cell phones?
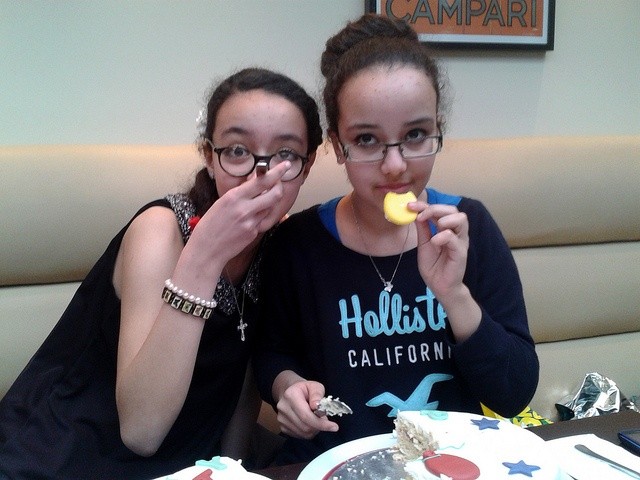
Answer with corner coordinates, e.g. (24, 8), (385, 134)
(617, 427), (640, 452)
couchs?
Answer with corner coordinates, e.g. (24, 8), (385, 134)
(0, 134), (639, 425)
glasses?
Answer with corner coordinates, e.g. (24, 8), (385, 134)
(333, 122), (443, 162)
(204, 139), (313, 184)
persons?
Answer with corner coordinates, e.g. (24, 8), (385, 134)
(248, 14), (540, 468)
(1, 65), (323, 480)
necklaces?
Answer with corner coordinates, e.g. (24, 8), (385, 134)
(223, 255), (258, 340)
(350, 191), (415, 294)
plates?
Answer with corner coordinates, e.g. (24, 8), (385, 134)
(295, 432), (555, 480)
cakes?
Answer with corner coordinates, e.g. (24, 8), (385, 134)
(389, 409), (566, 480)
(151, 455), (275, 480)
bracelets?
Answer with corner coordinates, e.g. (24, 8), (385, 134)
(161, 280), (217, 322)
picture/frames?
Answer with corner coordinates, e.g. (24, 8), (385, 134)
(364, 1), (556, 52)
